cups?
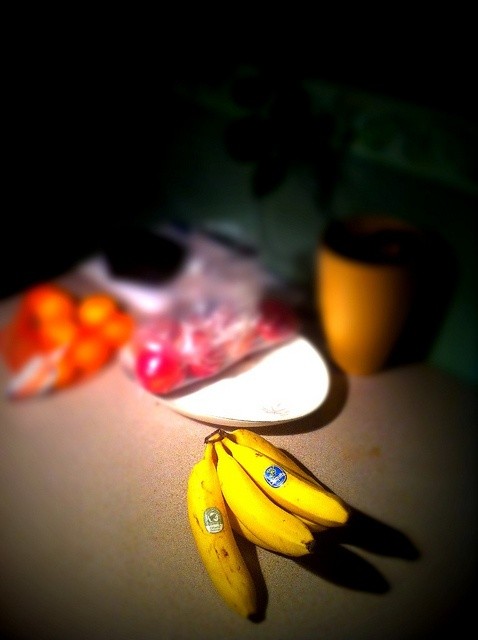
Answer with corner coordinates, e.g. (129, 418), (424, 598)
(315, 218), (418, 379)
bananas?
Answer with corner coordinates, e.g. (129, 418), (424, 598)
(188, 429), (350, 618)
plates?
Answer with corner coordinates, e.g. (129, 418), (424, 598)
(119, 333), (332, 428)
